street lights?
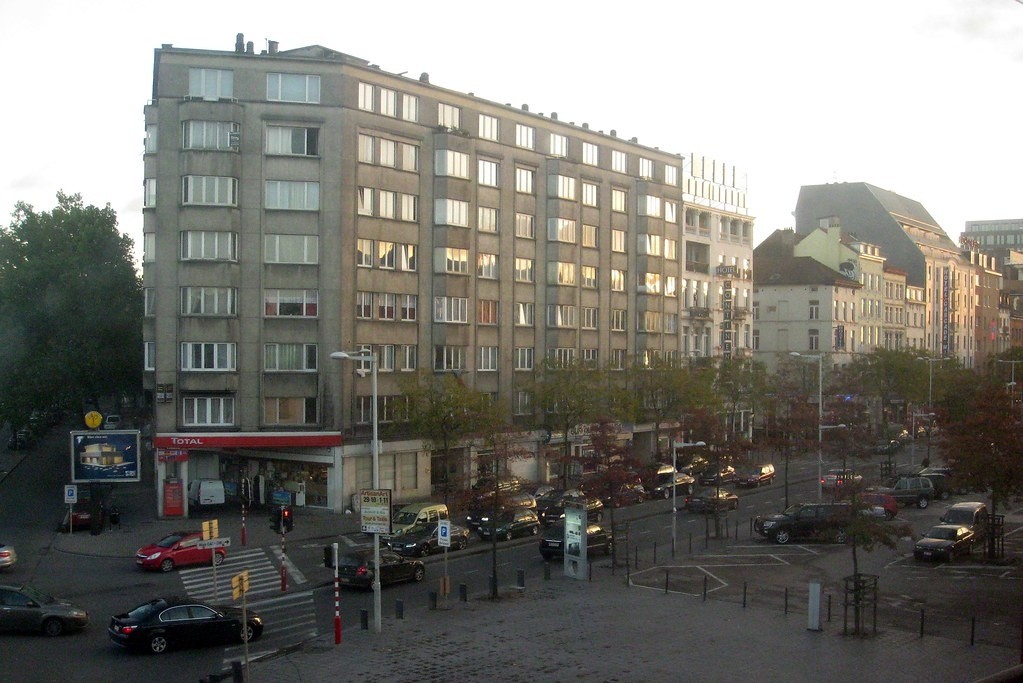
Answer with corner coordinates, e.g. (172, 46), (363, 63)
(329, 352), (382, 635)
(910, 412), (937, 474)
(789, 351), (846, 504)
(916, 357), (950, 412)
(997, 358), (1023, 409)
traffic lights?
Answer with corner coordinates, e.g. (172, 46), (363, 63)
(268, 505), (294, 535)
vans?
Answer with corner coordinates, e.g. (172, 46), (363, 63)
(381, 503), (450, 543)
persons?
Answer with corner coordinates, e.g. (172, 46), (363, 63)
(920, 455), (930, 467)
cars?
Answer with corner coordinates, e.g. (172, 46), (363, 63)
(1, 583), (88, 637)
(0, 542), (18, 567)
(672, 440), (706, 545)
(466, 424), (994, 565)
(539, 520), (616, 562)
(135, 530), (227, 572)
(104, 414), (124, 430)
(388, 522), (470, 558)
(6, 410), (49, 449)
(337, 550), (426, 592)
(109, 596), (266, 656)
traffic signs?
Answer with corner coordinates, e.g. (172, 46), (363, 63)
(196, 538), (231, 552)
